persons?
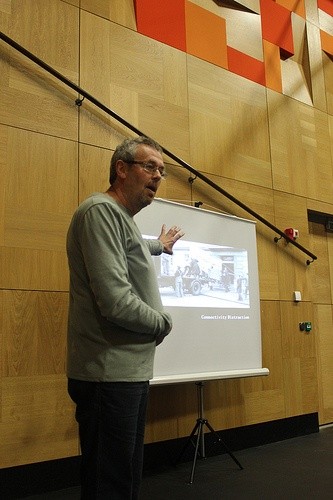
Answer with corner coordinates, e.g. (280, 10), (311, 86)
(64, 134), (185, 500)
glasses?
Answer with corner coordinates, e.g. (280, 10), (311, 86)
(122, 158), (166, 179)
